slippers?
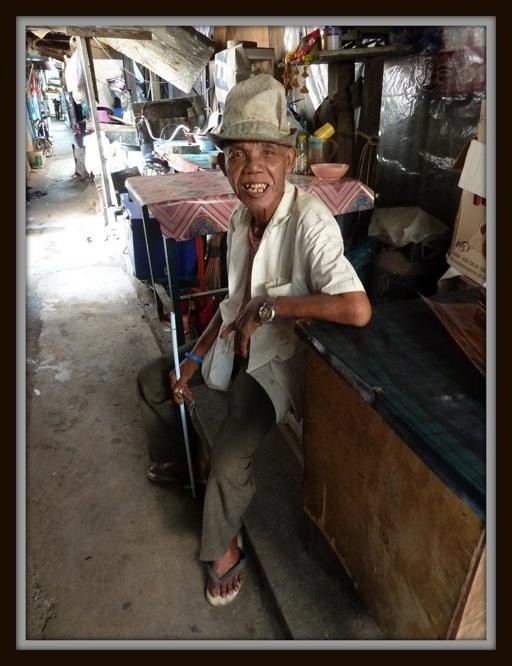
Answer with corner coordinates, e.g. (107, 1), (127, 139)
(144, 457), (185, 484)
(204, 529), (249, 606)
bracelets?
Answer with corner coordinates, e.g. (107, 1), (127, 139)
(184, 351), (204, 364)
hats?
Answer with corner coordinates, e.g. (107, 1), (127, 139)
(207, 75), (300, 151)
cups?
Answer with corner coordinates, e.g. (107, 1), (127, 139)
(315, 122), (335, 142)
(306, 138), (338, 175)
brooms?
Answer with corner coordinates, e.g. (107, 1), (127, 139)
(205, 232), (222, 290)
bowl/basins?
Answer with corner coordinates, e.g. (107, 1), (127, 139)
(185, 132), (216, 152)
(310, 162), (351, 181)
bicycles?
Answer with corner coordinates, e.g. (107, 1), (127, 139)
(133, 114), (191, 177)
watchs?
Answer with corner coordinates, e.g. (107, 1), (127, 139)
(257, 293), (279, 325)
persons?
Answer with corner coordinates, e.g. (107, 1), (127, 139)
(136, 74), (373, 611)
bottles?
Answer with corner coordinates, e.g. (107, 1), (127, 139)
(326, 26), (340, 51)
(294, 135), (308, 176)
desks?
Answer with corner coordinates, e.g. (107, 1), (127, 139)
(293, 298), (485, 642)
(125, 167), (370, 345)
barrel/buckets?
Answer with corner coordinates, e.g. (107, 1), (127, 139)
(26, 150), (45, 169)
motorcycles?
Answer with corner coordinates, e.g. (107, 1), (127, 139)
(32, 111), (53, 157)
